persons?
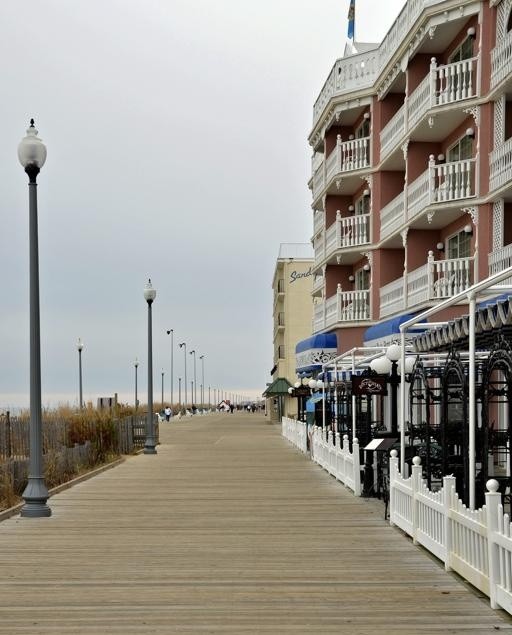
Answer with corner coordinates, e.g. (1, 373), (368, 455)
(164, 403), (265, 422)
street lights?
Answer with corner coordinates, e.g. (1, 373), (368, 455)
(199, 355), (205, 414)
(132, 357), (141, 413)
(288, 380), (334, 422)
(142, 277), (158, 454)
(166, 328), (174, 418)
(76, 337), (84, 415)
(179, 341), (188, 416)
(160, 368), (251, 422)
(15, 115), (54, 519)
(367, 343), (417, 438)
(190, 349), (197, 414)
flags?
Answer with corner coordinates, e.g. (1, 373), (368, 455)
(347, 0), (354, 39)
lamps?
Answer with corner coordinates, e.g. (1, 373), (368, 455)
(340, 22), (481, 287)
